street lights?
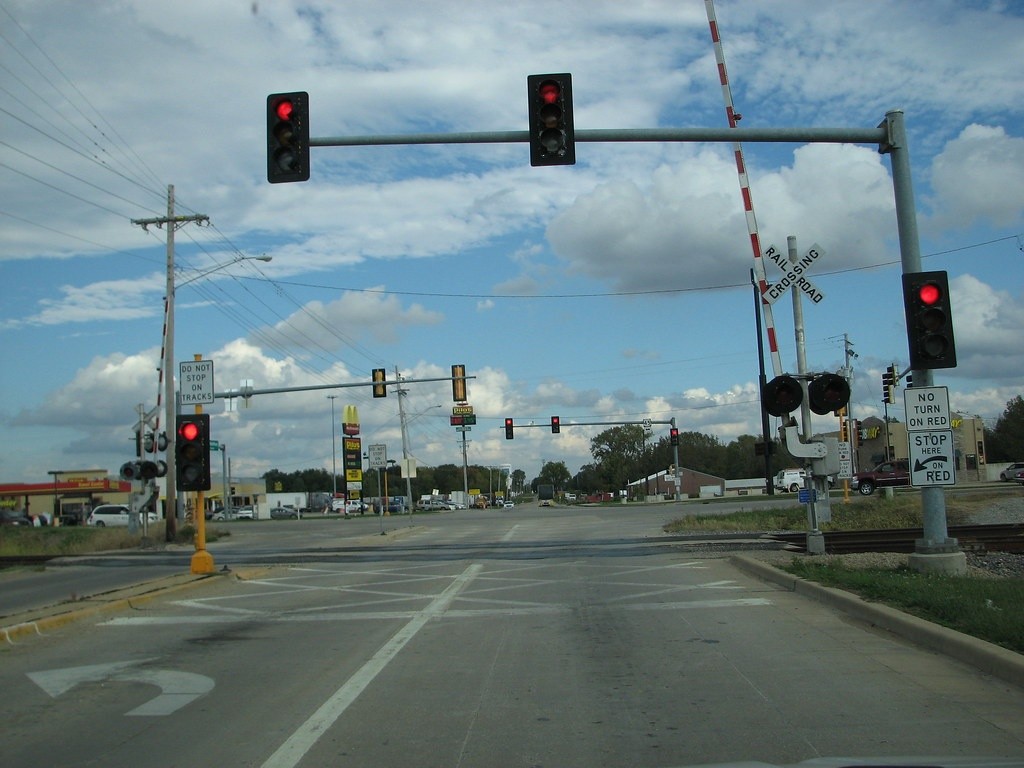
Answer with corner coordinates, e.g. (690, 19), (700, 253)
(163, 254), (273, 546)
(326, 394), (338, 500)
(401, 404), (442, 511)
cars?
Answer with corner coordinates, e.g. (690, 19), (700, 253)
(849, 457), (910, 495)
(204, 505), (254, 521)
(541, 499), (550, 506)
(331, 496), (504, 514)
(999, 463), (1024, 482)
(1012, 471), (1024, 486)
(271, 507), (303, 519)
(0, 508), (33, 529)
(503, 501), (515, 509)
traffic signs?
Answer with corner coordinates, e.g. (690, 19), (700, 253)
(907, 430), (957, 489)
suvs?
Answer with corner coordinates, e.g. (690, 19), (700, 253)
(775, 470), (836, 492)
(86, 504), (160, 527)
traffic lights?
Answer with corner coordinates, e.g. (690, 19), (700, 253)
(670, 428), (680, 446)
(763, 375), (803, 416)
(504, 418), (515, 440)
(123, 413), (210, 491)
(810, 374), (850, 412)
(901, 269), (958, 370)
(264, 91), (312, 184)
(528, 72), (578, 168)
(843, 421), (850, 443)
(881, 364), (915, 405)
(852, 418), (863, 450)
(551, 415), (560, 435)
